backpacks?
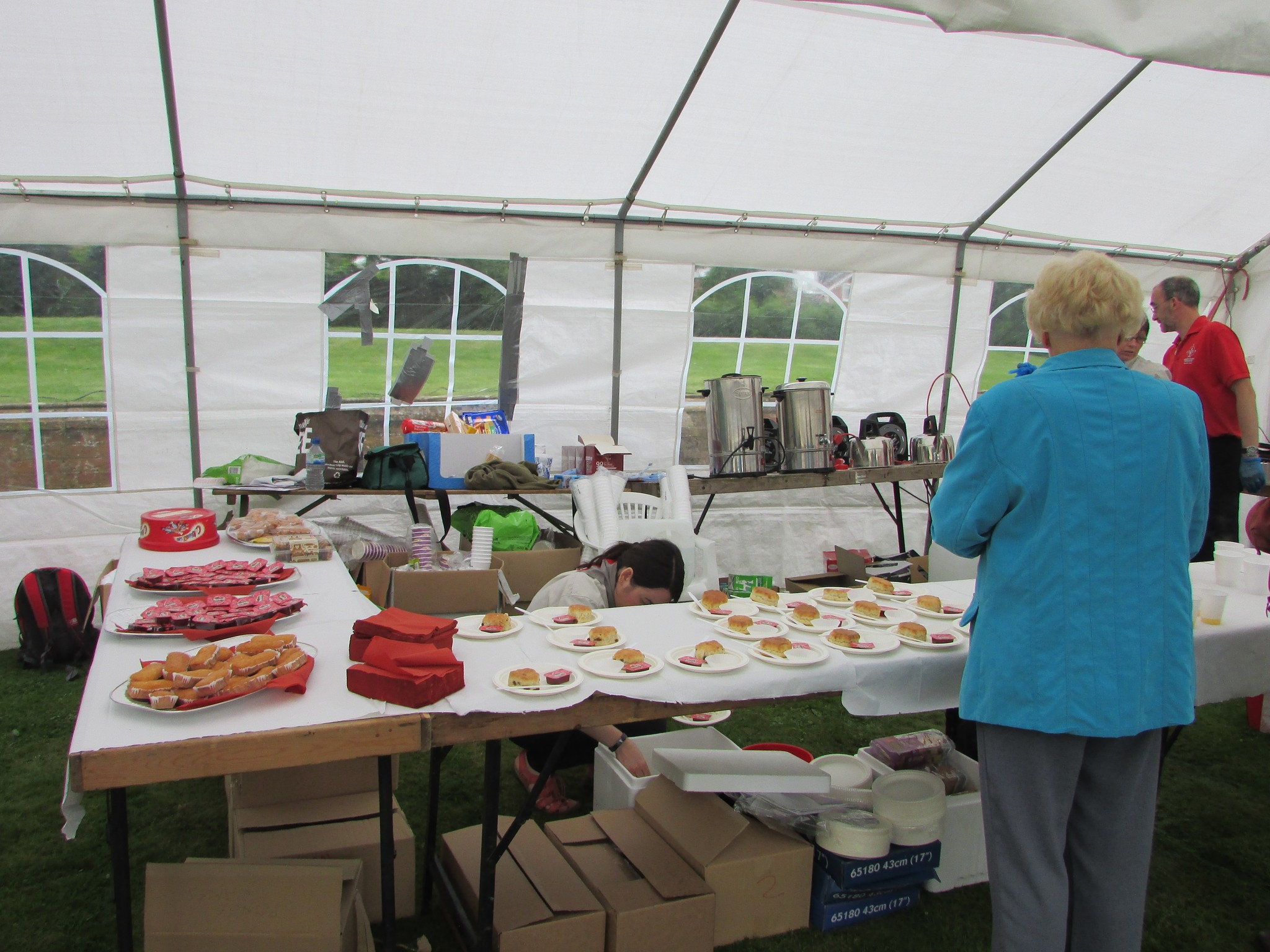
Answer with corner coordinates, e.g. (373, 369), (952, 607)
(12, 566), (96, 668)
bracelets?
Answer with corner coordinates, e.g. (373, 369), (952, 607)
(608, 733), (627, 752)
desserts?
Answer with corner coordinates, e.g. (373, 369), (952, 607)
(127, 634), (307, 709)
(229, 509), (333, 562)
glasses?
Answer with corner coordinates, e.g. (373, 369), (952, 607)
(1151, 296), (1182, 313)
(1124, 335), (1148, 344)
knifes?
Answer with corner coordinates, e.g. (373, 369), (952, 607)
(496, 578), (941, 690)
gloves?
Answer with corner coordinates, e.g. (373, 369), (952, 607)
(1008, 363), (1036, 377)
(1239, 457), (1267, 494)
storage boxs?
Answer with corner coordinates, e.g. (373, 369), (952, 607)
(272, 520), (583, 625)
(719, 545), (928, 599)
(143, 727), (987, 952)
(138, 507), (219, 552)
(83, 559), (120, 631)
(560, 433), (632, 493)
(403, 432), (535, 489)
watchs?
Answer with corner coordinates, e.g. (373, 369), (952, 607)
(1241, 445), (1259, 456)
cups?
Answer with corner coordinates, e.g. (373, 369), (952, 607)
(574, 465), (695, 569)
(1213, 541), (1270, 596)
(351, 525), (494, 571)
(532, 540), (554, 550)
(1190, 581), (1227, 628)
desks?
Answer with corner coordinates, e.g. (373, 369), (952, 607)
(212, 479), (572, 548)
(627, 459), (955, 551)
(63, 538), (435, 952)
(417, 560), (1270, 952)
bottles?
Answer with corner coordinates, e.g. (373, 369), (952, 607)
(305, 437), (325, 490)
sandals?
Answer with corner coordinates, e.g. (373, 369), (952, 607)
(513, 750), (570, 812)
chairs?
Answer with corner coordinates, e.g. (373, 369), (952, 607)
(574, 492), (720, 607)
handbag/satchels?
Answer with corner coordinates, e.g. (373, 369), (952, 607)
(293, 386), (370, 489)
(362, 442), (431, 492)
(201, 453), (296, 486)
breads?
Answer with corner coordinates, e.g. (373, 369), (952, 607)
(728, 615), (753, 634)
(567, 604), (592, 623)
(508, 668), (540, 690)
(694, 640), (725, 660)
(866, 577), (894, 595)
(760, 637), (792, 658)
(482, 613), (512, 631)
(750, 587), (779, 607)
(896, 621), (927, 641)
(700, 589), (727, 611)
(793, 604), (820, 626)
(916, 595), (942, 613)
(821, 588), (849, 602)
(612, 648), (644, 670)
(828, 628), (860, 647)
(587, 626), (619, 646)
(850, 601), (881, 620)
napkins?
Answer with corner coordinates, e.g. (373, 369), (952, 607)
(346, 606), (465, 709)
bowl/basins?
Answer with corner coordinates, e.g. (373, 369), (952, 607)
(741, 743), (813, 763)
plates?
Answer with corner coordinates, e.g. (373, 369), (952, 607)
(807, 587), (876, 607)
(845, 606), (918, 627)
(781, 610), (855, 633)
(672, 710), (731, 726)
(904, 598), (968, 619)
(103, 563), (320, 713)
(747, 593), (817, 613)
(546, 626), (626, 653)
(748, 639), (830, 666)
(493, 662), (584, 696)
(665, 644), (750, 674)
(713, 616), (789, 641)
(454, 614), (523, 639)
(528, 606), (603, 629)
(820, 629), (900, 655)
(952, 617), (969, 635)
(577, 649), (664, 679)
(687, 600), (759, 620)
(863, 581), (927, 601)
(886, 624), (963, 649)
(808, 754), (948, 860)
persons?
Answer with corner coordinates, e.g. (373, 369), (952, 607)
(929, 251), (1210, 950)
(1117, 313), (1172, 380)
(510, 540), (685, 813)
(1149, 276), (1266, 561)
